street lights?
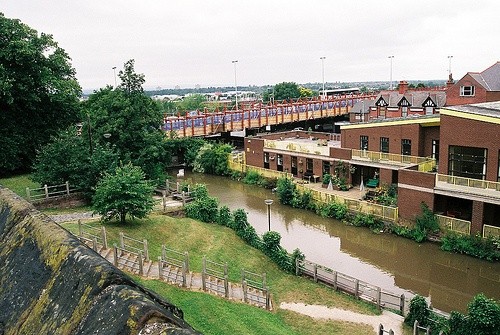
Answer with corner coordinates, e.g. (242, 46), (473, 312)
(265, 199), (274, 232)
(448, 56), (453, 75)
(232, 60), (239, 110)
(112, 67), (116, 89)
(388, 56), (395, 90)
(320, 56), (327, 99)
